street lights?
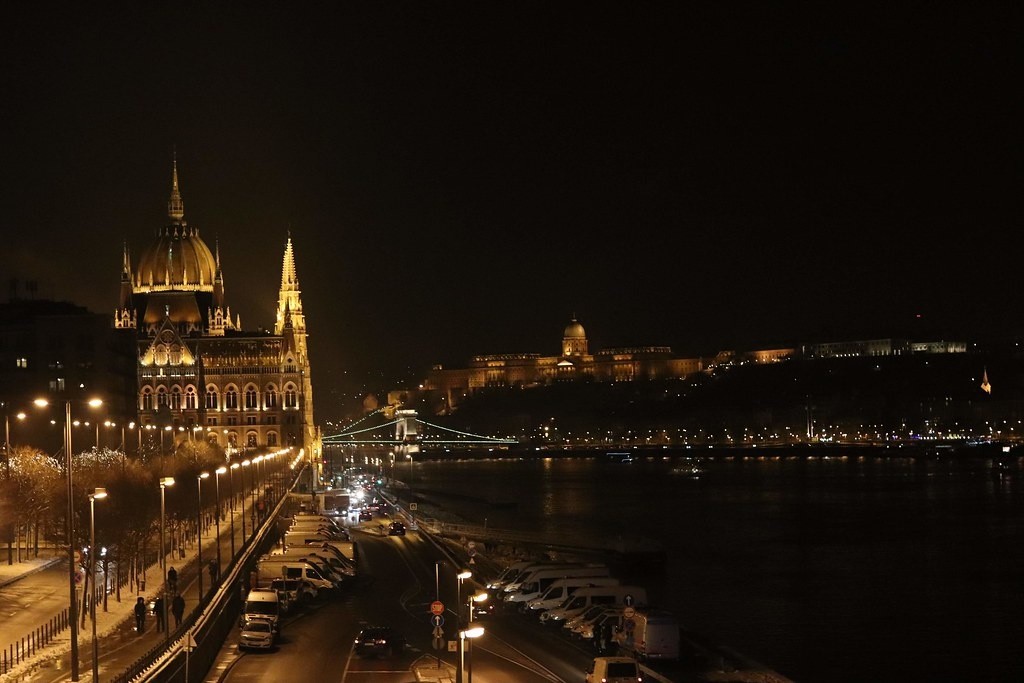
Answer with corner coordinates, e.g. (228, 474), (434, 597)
(290, 448), (305, 470)
(159, 477), (176, 642)
(111, 421), (210, 453)
(196, 472), (209, 603)
(214, 467), (227, 580)
(16, 399), (56, 425)
(229, 462), (240, 559)
(406, 454), (413, 478)
(456, 626), (486, 683)
(435, 560), (447, 600)
(241, 449), (290, 545)
(66, 398), (110, 683)
(389, 452), (396, 463)
(458, 571), (473, 605)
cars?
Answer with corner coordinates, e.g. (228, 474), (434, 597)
(335, 481), (394, 515)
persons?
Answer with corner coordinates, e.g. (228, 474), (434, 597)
(153, 595), (165, 633)
(168, 567), (177, 593)
(134, 597), (146, 632)
(592, 620), (613, 657)
(172, 590), (185, 628)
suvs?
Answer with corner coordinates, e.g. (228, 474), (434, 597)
(302, 578), (319, 602)
(355, 627), (406, 657)
(389, 522), (405, 535)
(464, 594), (495, 620)
(272, 579), (302, 607)
(359, 510), (372, 522)
(240, 619), (273, 650)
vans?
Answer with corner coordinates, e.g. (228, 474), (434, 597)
(248, 587), (279, 626)
(490, 557), (658, 641)
(622, 610), (681, 660)
(258, 515), (358, 587)
(585, 657), (642, 683)
